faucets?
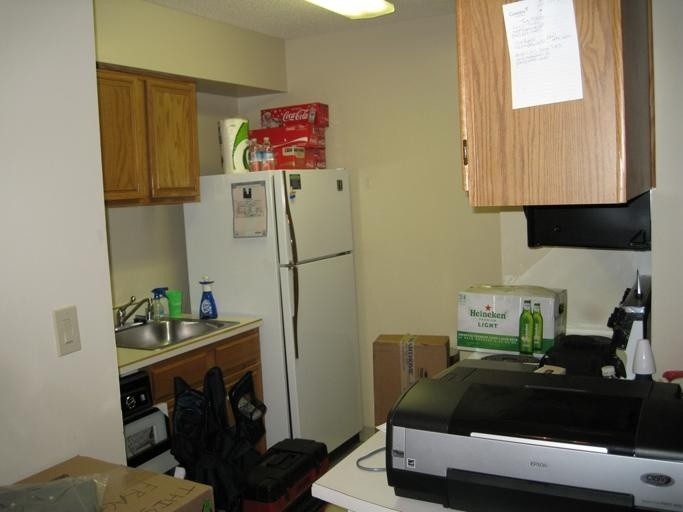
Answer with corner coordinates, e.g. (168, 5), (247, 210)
(117, 296), (152, 327)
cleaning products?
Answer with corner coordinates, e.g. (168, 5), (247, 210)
(150, 288), (169, 317)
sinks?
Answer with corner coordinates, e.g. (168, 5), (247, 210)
(114, 318), (240, 350)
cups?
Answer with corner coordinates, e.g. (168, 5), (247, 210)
(166, 289), (185, 320)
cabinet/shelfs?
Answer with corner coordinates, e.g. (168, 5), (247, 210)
(95, 68), (200, 202)
(148, 332), (267, 454)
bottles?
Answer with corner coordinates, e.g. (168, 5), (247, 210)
(519, 300), (543, 354)
(247, 136), (274, 172)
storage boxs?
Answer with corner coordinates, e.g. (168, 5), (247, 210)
(370, 281), (569, 433)
(14, 455), (213, 512)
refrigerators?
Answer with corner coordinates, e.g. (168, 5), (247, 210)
(182, 169), (363, 455)
